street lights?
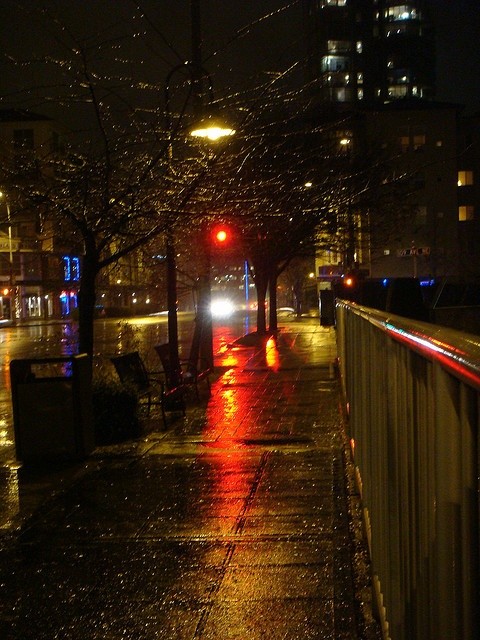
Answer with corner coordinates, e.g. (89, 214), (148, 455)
(0, 192), (14, 322)
(339, 132), (355, 273)
(158, 63), (237, 378)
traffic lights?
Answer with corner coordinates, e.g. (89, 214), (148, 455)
(212, 226), (232, 248)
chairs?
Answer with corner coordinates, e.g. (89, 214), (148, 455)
(154, 342), (212, 397)
(109, 351), (195, 431)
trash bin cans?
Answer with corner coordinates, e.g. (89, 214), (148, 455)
(10, 354), (96, 462)
